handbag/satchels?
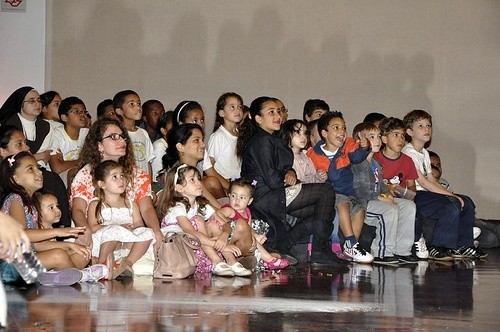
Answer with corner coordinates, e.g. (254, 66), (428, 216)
(152, 230), (202, 280)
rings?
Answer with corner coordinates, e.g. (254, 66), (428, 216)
(16, 242), (23, 247)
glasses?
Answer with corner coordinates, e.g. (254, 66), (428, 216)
(67, 110), (89, 115)
(99, 132), (128, 141)
(23, 99), (44, 104)
(281, 108), (288, 113)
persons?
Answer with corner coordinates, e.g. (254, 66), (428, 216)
(237, 96), (350, 268)
(0, 86), (488, 287)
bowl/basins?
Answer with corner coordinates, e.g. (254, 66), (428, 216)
(395, 185), (416, 200)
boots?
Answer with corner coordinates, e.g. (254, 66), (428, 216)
(310, 218), (350, 268)
(271, 221), (309, 266)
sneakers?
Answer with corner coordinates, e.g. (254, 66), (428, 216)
(414, 236), (430, 259)
(428, 245), (488, 261)
(37, 267), (83, 288)
(80, 263), (109, 283)
(373, 253), (420, 264)
(342, 239), (374, 263)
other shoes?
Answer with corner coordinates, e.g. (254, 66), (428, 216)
(211, 253), (290, 277)
(101, 259), (134, 277)
